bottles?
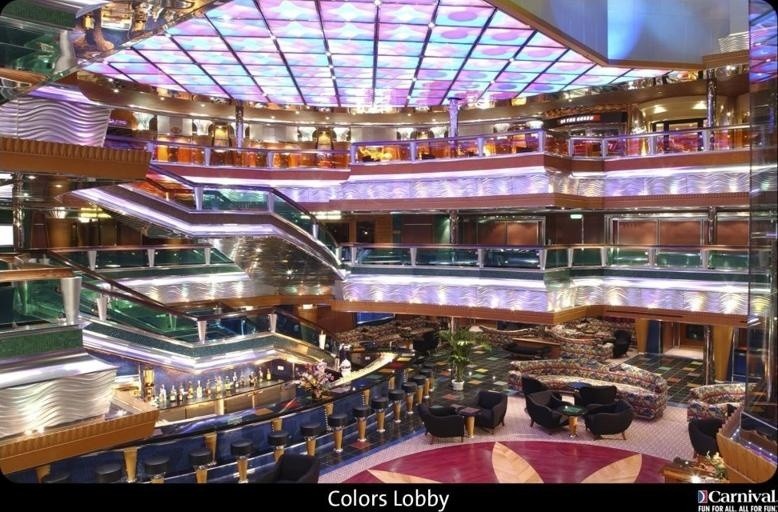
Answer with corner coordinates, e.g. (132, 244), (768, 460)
(160, 366), (272, 402)
